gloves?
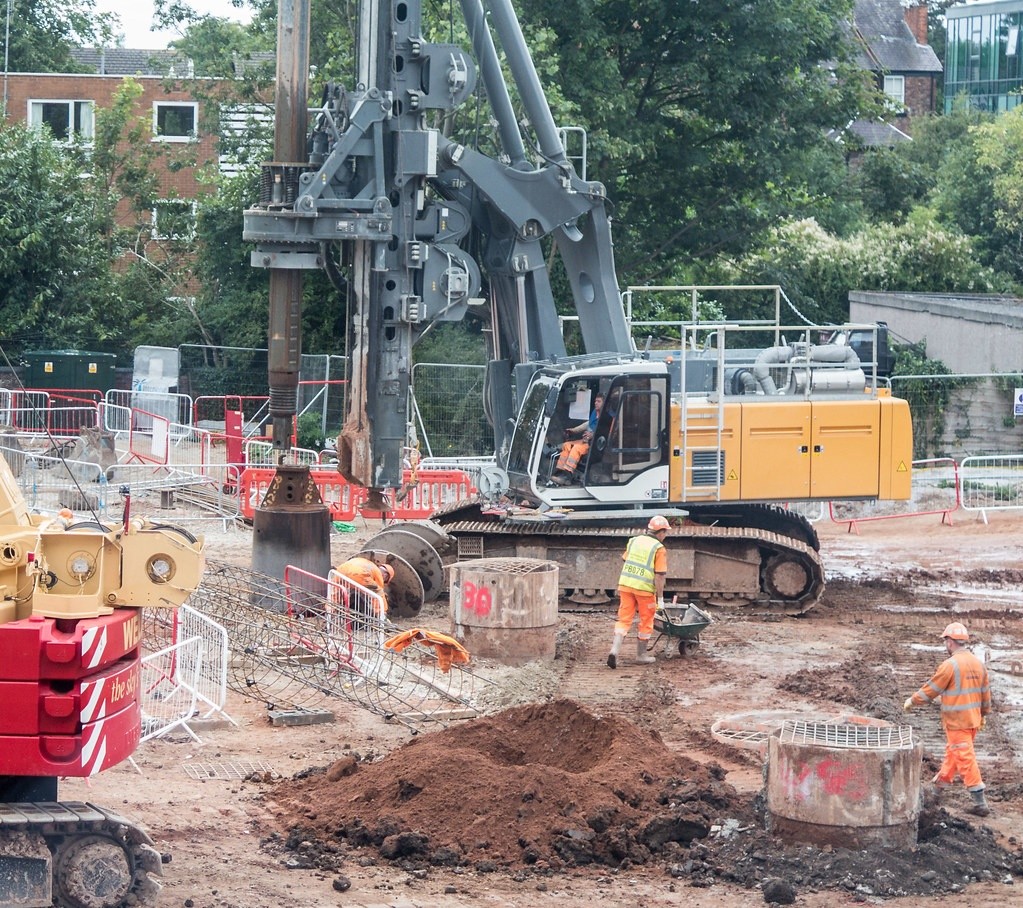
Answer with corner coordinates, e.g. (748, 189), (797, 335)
(903, 698), (914, 712)
(980, 716), (987, 730)
(656, 597), (664, 611)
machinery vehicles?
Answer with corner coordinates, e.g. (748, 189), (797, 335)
(2, 446), (204, 908)
(419, 284), (914, 625)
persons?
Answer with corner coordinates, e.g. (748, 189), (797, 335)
(322, 554), (400, 641)
(605, 512), (672, 667)
(547, 391), (622, 489)
(904, 617), (994, 814)
(60, 506), (74, 524)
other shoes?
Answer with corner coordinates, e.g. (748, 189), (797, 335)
(551, 467), (571, 488)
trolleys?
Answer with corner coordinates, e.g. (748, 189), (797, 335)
(634, 604), (712, 659)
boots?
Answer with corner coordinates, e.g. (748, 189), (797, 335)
(636, 637), (654, 665)
(606, 635), (624, 669)
(922, 780), (940, 802)
(962, 790), (988, 814)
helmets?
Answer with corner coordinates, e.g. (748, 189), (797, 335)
(58, 508), (71, 520)
(647, 516), (672, 532)
(940, 622), (969, 643)
(381, 562), (394, 585)
